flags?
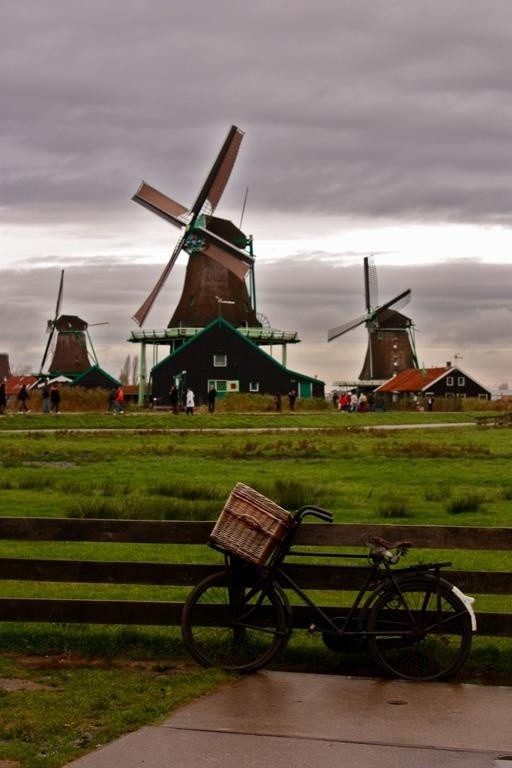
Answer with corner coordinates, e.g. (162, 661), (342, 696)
(455, 353), (462, 359)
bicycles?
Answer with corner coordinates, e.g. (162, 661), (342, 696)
(180, 482), (478, 681)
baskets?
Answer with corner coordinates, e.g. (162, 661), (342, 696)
(209, 481), (294, 566)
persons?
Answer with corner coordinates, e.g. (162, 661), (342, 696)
(428, 395), (434, 411)
(104, 385), (124, 415)
(288, 389), (297, 410)
(0, 384), (8, 415)
(167, 384), (178, 415)
(49, 384), (61, 414)
(185, 386), (195, 415)
(332, 388), (373, 412)
(41, 380), (50, 413)
(207, 384), (218, 413)
(17, 384), (32, 413)
